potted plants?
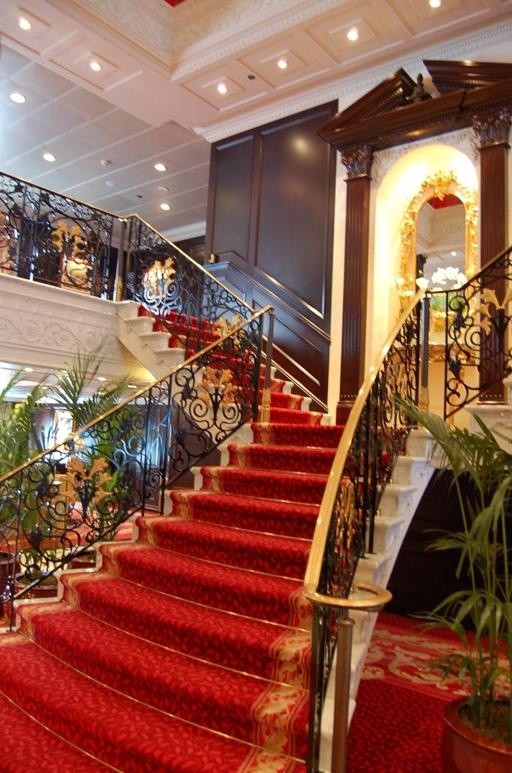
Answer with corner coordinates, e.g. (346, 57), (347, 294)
(396, 386), (512, 772)
(0, 360), (143, 603)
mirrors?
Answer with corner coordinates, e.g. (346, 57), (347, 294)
(399, 168), (479, 333)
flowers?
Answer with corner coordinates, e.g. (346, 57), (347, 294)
(414, 264), (471, 315)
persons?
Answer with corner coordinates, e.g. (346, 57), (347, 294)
(413, 73), (424, 104)
